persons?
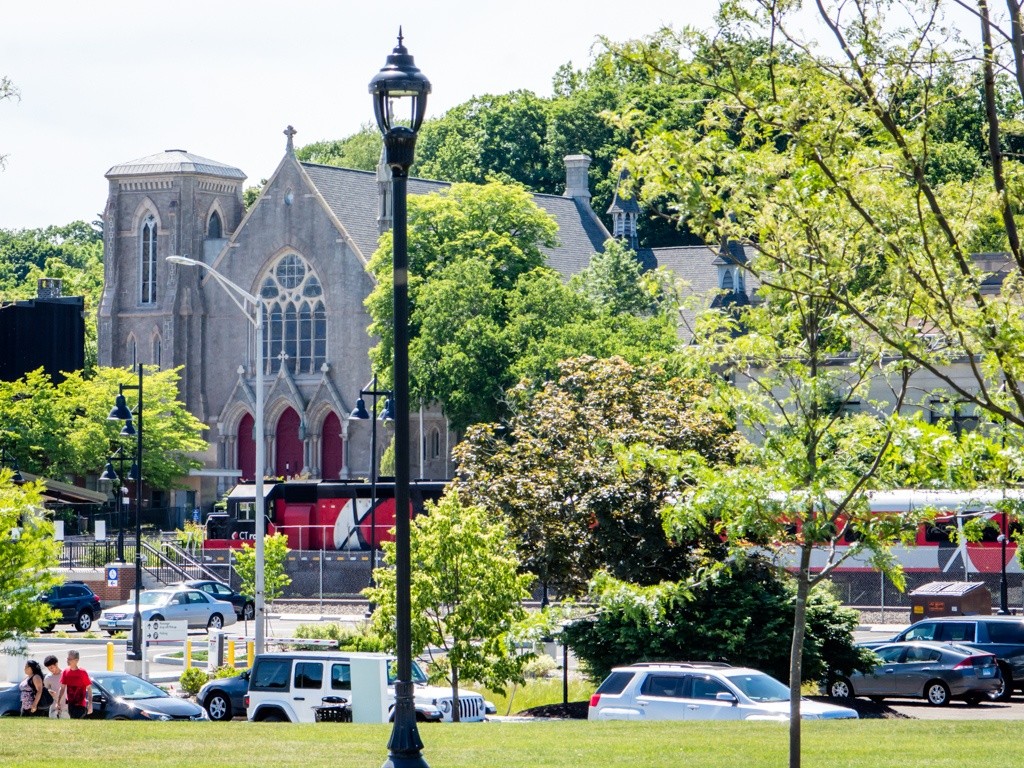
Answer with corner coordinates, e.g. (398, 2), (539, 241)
(19, 660), (44, 717)
(55, 650), (93, 719)
(43, 655), (70, 719)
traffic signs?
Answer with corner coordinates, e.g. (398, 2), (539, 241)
(140, 620), (189, 643)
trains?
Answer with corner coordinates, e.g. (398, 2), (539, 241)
(197, 479), (1024, 615)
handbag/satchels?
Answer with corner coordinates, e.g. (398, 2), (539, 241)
(27, 675), (54, 707)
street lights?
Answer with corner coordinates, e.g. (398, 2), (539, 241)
(103, 442), (139, 561)
(105, 363), (146, 662)
(348, 373), (396, 618)
(164, 255), (265, 655)
(995, 379), (1012, 616)
(364, 25), (434, 768)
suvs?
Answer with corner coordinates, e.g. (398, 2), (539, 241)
(587, 660), (860, 724)
(850, 615), (1024, 703)
(243, 650), (497, 724)
(5, 579), (104, 633)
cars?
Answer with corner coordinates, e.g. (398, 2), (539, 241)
(168, 579), (255, 621)
(195, 663), (254, 723)
(96, 586), (238, 636)
(0, 671), (210, 723)
(817, 640), (1004, 708)
